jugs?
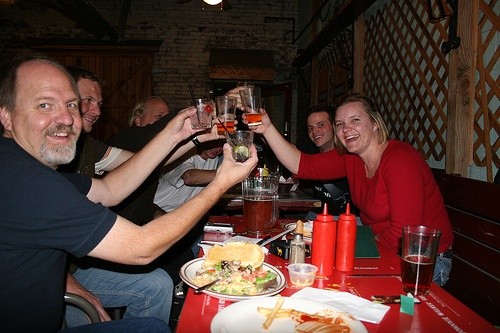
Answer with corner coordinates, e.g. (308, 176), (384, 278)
(242, 176), (279, 235)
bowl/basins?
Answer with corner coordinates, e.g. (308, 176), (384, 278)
(278, 183), (294, 193)
(286, 262), (319, 287)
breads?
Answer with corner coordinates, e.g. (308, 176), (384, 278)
(207, 242), (265, 269)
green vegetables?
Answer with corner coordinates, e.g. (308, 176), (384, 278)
(213, 261), (276, 293)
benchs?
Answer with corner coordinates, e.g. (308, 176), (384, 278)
(430, 170), (500, 333)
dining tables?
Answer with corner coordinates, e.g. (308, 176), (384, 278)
(174, 188), (500, 333)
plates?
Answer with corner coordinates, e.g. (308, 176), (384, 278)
(284, 223), (297, 234)
(179, 257), (286, 302)
(212, 296), (370, 333)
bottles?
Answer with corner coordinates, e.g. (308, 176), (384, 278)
(311, 202), (337, 276)
(335, 202), (358, 272)
(289, 233), (305, 264)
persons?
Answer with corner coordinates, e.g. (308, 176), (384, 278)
(107, 82), (253, 283)
(62, 72), (238, 329)
(262, 105), (361, 220)
(0, 53), (258, 333)
(241, 93), (453, 289)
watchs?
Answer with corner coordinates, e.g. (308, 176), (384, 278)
(191, 136), (201, 146)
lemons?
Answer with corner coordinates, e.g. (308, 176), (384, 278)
(233, 145), (249, 157)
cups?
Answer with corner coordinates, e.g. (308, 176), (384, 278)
(216, 96), (238, 134)
(401, 225), (442, 294)
(188, 99), (214, 129)
(239, 87), (262, 126)
(225, 130), (254, 162)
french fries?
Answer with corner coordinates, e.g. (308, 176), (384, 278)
(257, 298), (318, 329)
